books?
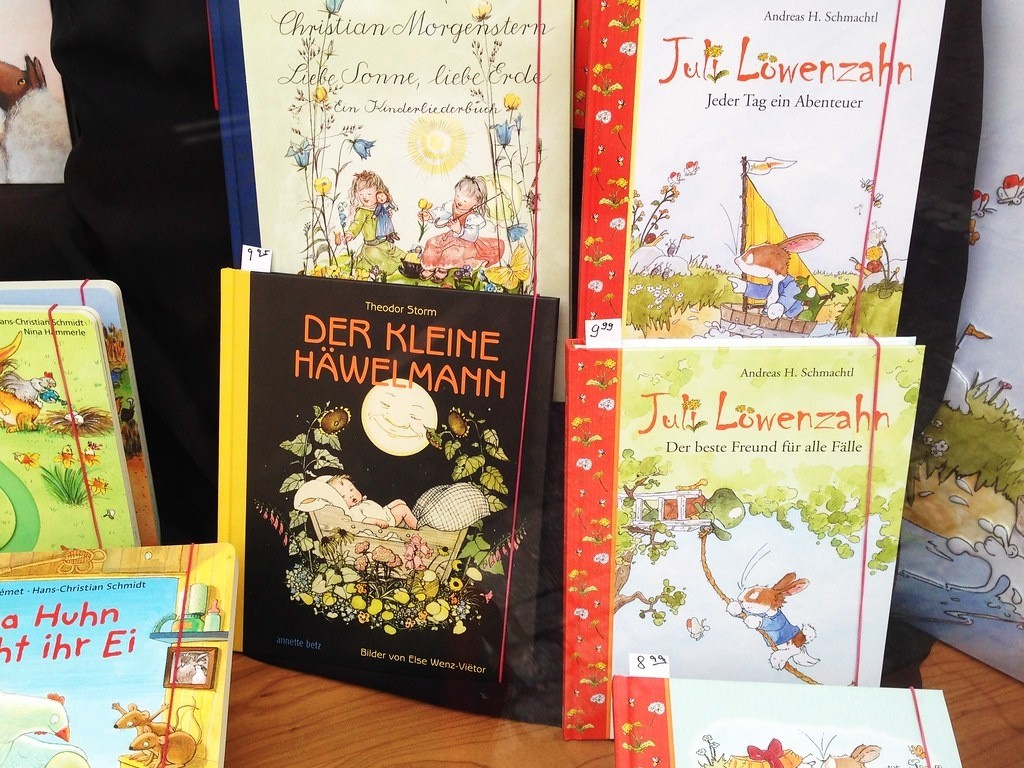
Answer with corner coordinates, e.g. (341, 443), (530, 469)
(207, 0), (575, 402)
(0, 304), (140, 552)
(575, 2), (947, 347)
(216, 267), (562, 727)
(0, 541), (238, 768)
(0, 280), (161, 547)
(891, 2), (1024, 688)
(561, 338), (961, 767)
